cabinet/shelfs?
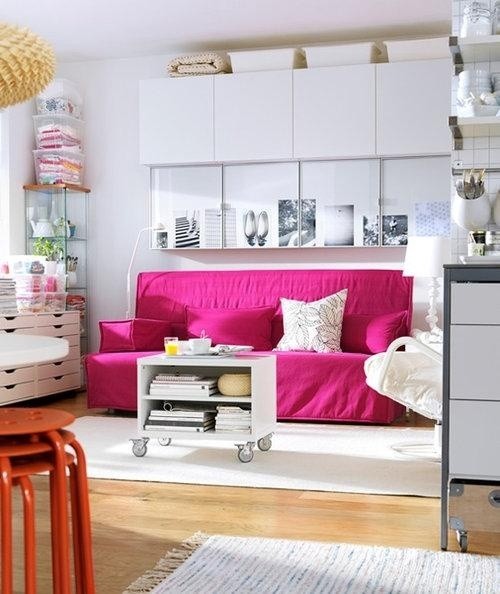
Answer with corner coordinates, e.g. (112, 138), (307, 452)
(136, 64), (294, 169)
(146, 159), (301, 251)
(131, 349), (277, 463)
(294, 57), (451, 161)
(301, 150), (453, 253)
(445, 35), (500, 142)
(21, 181), (91, 358)
(1, 307), (86, 408)
(437, 257), (500, 555)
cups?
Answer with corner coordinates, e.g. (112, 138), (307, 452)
(163, 336), (178, 356)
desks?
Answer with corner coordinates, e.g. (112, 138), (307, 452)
(0, 331), (72, 372)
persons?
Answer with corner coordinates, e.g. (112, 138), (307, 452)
(389, 218), (398, 232)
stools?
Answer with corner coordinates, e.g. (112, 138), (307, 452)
(1, 403), (98, 594)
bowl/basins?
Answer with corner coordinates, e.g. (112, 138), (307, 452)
(189, 339), (211, 353)
(456, 68), (500, 116)
(460, 1), (500, 39)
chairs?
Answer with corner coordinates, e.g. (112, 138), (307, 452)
(361, 336), (442, 460)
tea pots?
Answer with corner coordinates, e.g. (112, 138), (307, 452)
(31, 219), (54, 237)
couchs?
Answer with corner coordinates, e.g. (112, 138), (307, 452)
(83, 267), (416, 427)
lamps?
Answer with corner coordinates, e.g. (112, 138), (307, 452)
(396, 232), (453, 344)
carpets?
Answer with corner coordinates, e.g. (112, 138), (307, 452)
(119, 527), (500, 594)
(30, 409), (443, 500)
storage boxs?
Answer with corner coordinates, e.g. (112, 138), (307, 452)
(31, 77), (88, 187)
(226, 31), (451, 76)
(10, 251), (69, 315)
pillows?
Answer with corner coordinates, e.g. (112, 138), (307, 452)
(340, 308), (412, 358)
(270, 286), (350, 355)
(97, 317), (172, 353)
(183, 302), (277, 351)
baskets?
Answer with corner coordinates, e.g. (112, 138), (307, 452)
(218, 374), (251, 396)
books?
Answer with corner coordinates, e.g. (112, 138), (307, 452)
(144, 372), (252, 436)
(0, 278), (18, 317)
